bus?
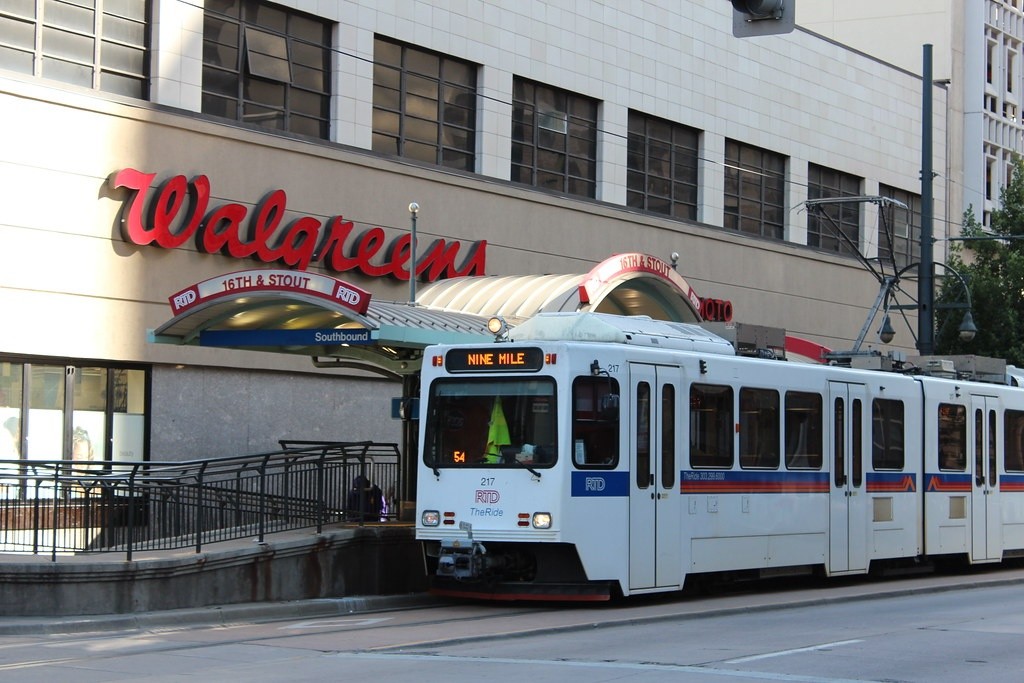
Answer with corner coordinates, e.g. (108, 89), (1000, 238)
(393, 306), (1024, 604)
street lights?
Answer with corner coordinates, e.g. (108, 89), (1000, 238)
(878, 259), (977, 352)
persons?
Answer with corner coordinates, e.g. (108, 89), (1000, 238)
(347, 476), (384, 523)
(72, 427), (101, 498)
